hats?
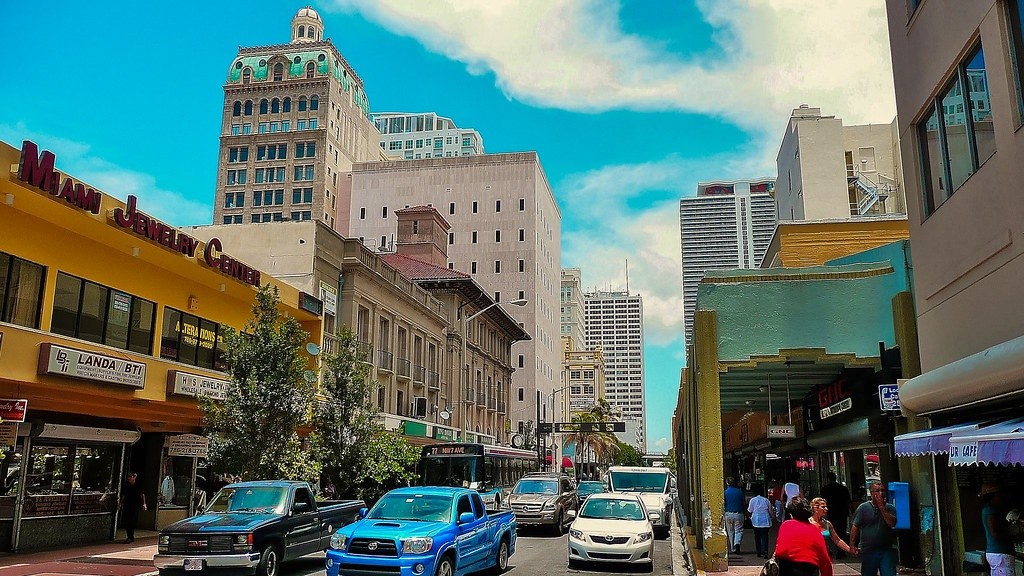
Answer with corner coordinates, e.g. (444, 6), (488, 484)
(978, 483), (1000, 496)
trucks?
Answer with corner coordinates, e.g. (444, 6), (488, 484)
(608, 466), (676, 536)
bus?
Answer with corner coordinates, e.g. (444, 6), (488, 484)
(419, 443), (539, 513)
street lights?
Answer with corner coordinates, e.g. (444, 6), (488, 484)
(462, 298), (529, 444)
(550, 383), (591, 473)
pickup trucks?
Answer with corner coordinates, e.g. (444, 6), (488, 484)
(153, 480), (367, 576)
(325, 486), (517, 576)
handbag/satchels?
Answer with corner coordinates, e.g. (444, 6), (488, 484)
(759, 552), (780, 576)
(775, 499), (784, 523)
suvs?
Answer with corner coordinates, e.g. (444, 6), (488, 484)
(498, 471), (580, 537)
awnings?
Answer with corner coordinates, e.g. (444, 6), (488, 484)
(893, 417), (1001, 457)
(948, 416), (1024, 467)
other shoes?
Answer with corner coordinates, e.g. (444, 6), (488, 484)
(125, 538), (130, 544)
(733, 551), (737, 554)
(736, 545), (740, 554)
(130, 536), (134, 542)
(764, 554), (767, 559)
(761, 554), (764, 557)
(758, 554), (761, 557)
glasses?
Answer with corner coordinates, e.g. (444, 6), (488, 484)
(871, 489), (886, 492)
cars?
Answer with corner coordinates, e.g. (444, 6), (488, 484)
(567, 492), (660, 572)
(576, 480), (610, 505)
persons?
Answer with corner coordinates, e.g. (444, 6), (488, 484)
(775, 496), (833, 576)
(979, 486), (1024, 576)
(850, 482), (897, 576)
(821, 472), (851, 558)
(724, 472), (813, 559)
(121, 471), (154, 549)
(807, 497), (859, 576)
